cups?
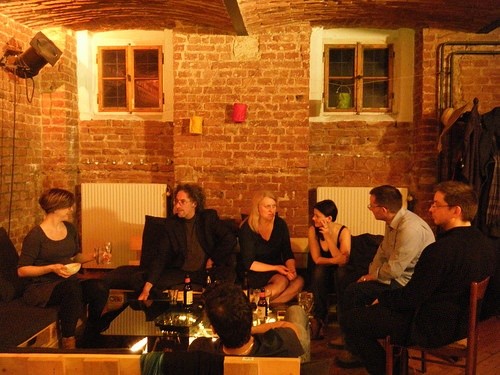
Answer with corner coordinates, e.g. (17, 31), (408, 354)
(277, 311), (286, 321)
(299, 293), (314, 313)
(232, 103), (247, 122)
(168, 289), (178, 305)
(189, 117), (203, 134)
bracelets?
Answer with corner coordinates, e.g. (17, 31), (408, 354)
(360, 276), (367, 282)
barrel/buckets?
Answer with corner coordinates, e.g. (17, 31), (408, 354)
(336, 85), (351, 109)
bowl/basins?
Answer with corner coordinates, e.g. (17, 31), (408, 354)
(61, 263), (82, 275)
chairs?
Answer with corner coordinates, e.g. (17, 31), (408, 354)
(384, 271), (500, 375)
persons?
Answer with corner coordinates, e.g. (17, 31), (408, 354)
(326, 185), (435, 368)
(188, 282), (309, 363)
(307, 199), (351, 340)
(340, 180), (500, 375)
(16, 188), (110, 350)
(130, 183), (240, 300)
(239, 189), (304, 304)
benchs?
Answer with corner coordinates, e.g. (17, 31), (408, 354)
(100, 214), (168, 312)
(0, 345), (301, 375)
(0, 226), (89, 349)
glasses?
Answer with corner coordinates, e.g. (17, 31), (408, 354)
(367, 204), (386, 211)
(174, 198), (192, 205)
(431, 201), (456, 208)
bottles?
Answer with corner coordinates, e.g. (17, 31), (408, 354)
(183, 274), (193, 305)
(257, 289), (268, 320)
(249, 288), (257, 326)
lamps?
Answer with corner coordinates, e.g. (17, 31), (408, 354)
(0, 31), (63, 79)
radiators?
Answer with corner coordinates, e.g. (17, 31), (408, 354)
(80, 182), (167, 270)
(316, 186), (408, 237)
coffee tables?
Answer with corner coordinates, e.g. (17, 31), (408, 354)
(98, 292), (218, 354)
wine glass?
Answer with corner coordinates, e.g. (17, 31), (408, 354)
(104, 242), (112, 264)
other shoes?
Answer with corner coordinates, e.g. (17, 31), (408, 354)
(334, 348), (365, 367)
(327, 336), (346, 348)
(311, 319), (324, 339)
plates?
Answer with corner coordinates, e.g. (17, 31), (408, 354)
(155, 308), (203, 334)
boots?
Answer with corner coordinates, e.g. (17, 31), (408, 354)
(85, 320), (108, 344)
(61, 335), (77, 350)
